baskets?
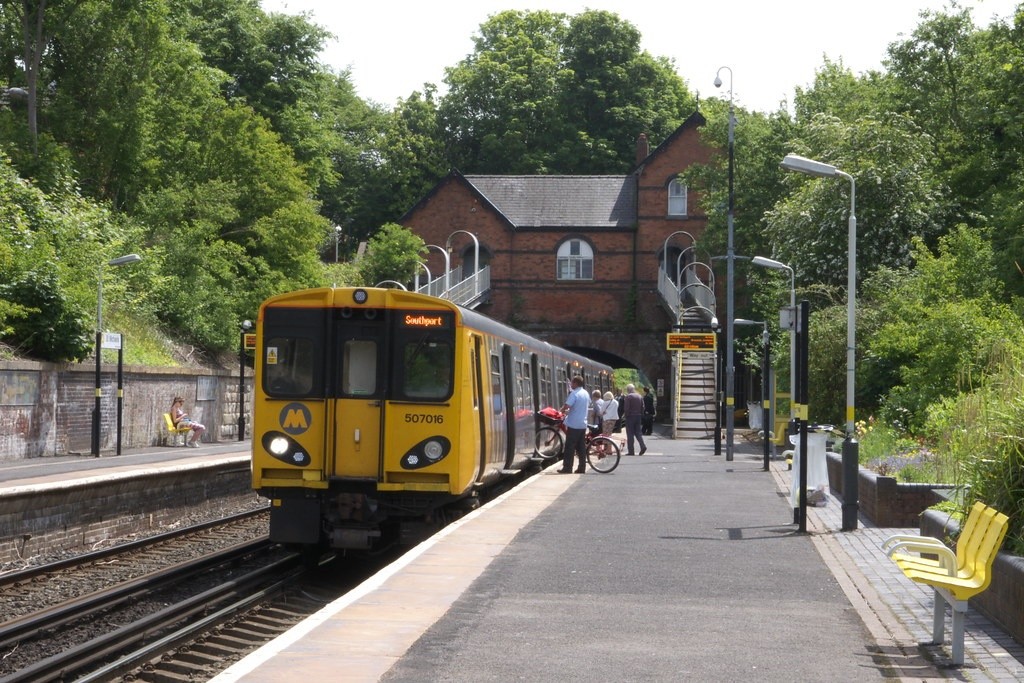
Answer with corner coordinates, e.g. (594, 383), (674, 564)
(537, 407), (563, 425)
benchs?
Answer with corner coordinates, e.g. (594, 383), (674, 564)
(758, 424), (786, 460)
(882, 503), (1009, 665)
(163, 413), (190, 447)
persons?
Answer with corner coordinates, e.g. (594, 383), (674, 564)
(613, 387), (654, 435)
(623, 383), (647, 455)
(592, 390), (619, 455)
(172, 396), (205, 448)
(556, 376), (591, 473)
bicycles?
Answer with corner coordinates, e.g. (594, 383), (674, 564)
(533, 409), (621, 474)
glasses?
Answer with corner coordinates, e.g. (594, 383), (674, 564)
(180, 401), (182, 406)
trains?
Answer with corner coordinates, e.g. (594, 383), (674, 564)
(252, 287), (618, 560)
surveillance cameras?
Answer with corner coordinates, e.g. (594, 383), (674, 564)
(714, 79), (722, 87)
(711, 317), (718, 328)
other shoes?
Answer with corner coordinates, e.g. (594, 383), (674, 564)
(557, 469), (572, 473)
(606, 452), (612, 455)
(575, 469), (586, 473)
(639, 446), (647, 455)
(626, 453), (634, 456)
(187, 441), (201, 448)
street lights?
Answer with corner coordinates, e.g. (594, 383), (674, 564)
(733, 316), (768, 473)
(714, 65), (735, 462)
(90, 252), (142, 458)
(750, 255), (797, 471)
(779, 152), (860, 532)
